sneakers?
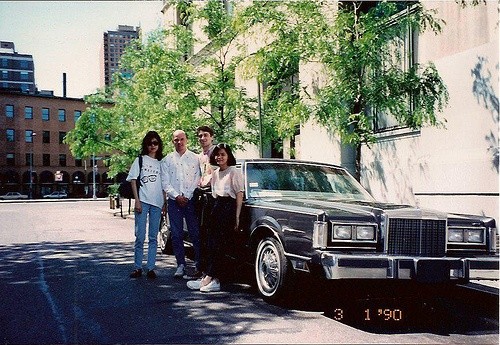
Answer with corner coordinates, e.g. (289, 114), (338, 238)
(200, 280), (220, 292)
(186, 277), (205, 289)
(174, 264), (186, 277)
(183, 271), (203, 280)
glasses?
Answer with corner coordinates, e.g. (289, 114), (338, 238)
(147, 141), (160, 146)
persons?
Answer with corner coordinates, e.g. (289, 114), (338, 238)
(188, 143), (246, 295)
(158, 129), (201, 276)
(194, 126), (222, 272)
(126, 131), (166, 278)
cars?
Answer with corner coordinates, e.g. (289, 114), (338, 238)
(156, 157), (499, 310)
(0, 191), (28, 200)
(44, 191), (68, 199)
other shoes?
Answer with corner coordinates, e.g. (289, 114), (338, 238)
(130, 269), (142, 278)
(147, 271), (156, 279)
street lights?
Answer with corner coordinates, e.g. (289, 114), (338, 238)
(30, 132), (37, 197)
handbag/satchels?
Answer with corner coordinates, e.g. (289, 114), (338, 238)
(118, 153), (143, 199)
